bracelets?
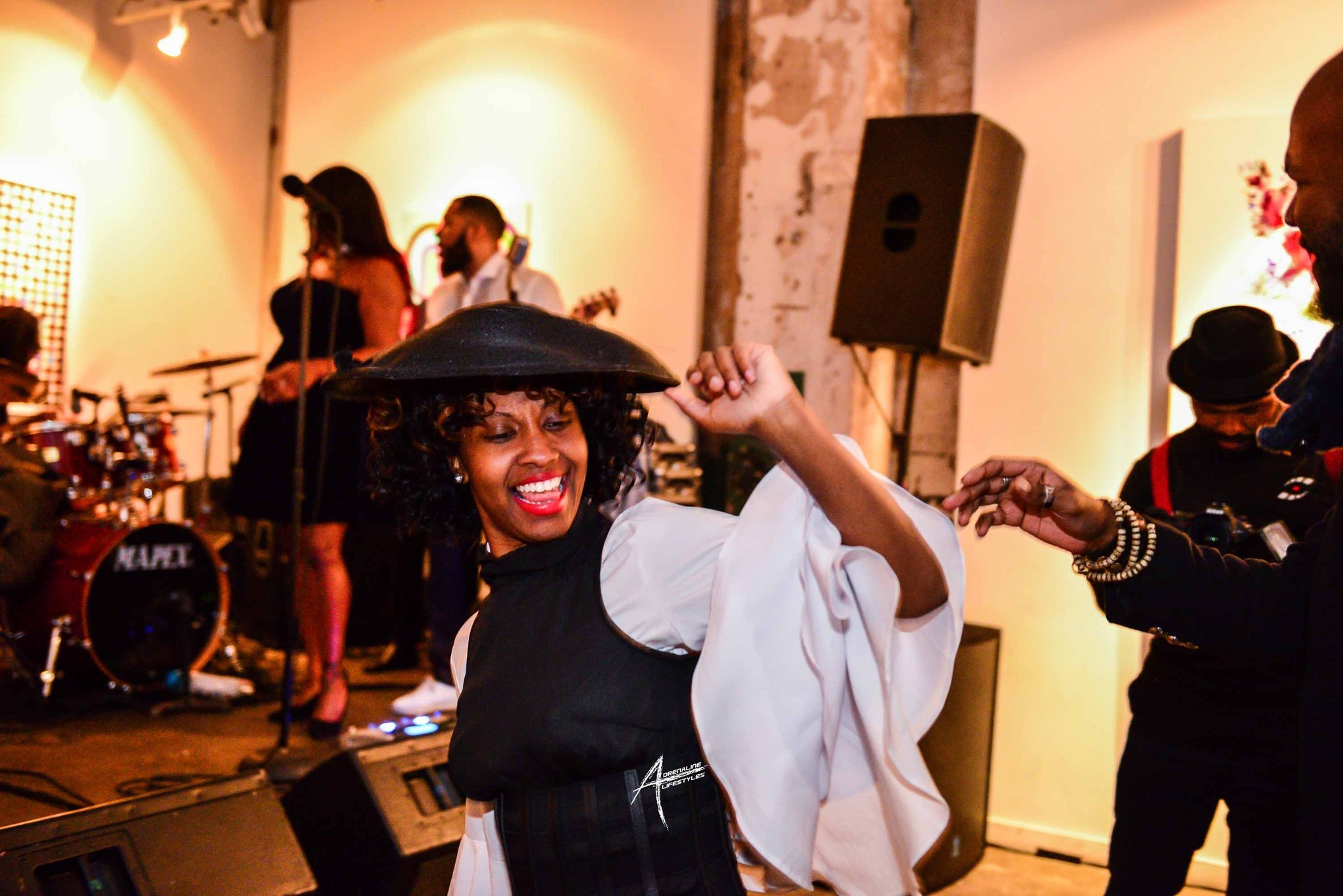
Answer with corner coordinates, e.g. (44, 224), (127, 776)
(1074, 499), (1156, 580)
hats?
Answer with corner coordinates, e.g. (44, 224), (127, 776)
(1165, 306), (1300, 406)
(354, 299), (680, 393)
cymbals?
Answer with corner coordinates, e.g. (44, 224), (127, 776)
(151, 355), (260, 375)
(126, 409), (207, 416)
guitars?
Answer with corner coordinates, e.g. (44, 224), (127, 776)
(568, 286), (621, 324)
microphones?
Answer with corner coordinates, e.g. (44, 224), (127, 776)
(280, 174), (342, 217)
(511, 238), (526, 265)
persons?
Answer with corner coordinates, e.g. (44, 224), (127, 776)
(1104, 306), (1324, 896)
(942, 46), (1343, 896)
(315, 300), (965, 896)
(224, 165), (564, 738)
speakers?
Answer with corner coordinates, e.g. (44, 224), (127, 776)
(912, 625), (1002, 895)
(830, 110), (1025, 367)
(288, 723), (496, 895)
(1, 765), (318, 895)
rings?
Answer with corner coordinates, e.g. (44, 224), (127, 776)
(1003, 477), (1008, 491)
(1043, 483), (1054, 505)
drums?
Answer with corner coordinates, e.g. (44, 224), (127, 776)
(5, 418), (93, 513)
(2, 430), (97, 590)
(96, 417), (186, 500)
(48, 518), (228, 694)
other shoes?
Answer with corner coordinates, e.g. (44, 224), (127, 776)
(309, 691), (349, 740)
(390, 677), (458, 716)
(268, 695), (318, 722)
(365, 642), (420, 673)
